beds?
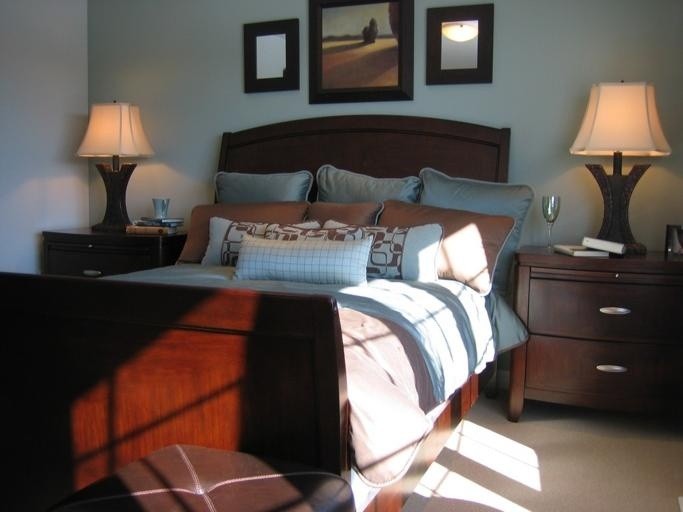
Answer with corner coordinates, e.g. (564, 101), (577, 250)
(0, 114), (510, 512)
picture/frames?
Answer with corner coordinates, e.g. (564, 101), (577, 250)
(307, 0), (414, 105)
(244, 17), (300, 94)
(425, 2), (495, 86)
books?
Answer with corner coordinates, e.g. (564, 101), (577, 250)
(126, 217), (184, 235)
(582, 236), (624, 255)
(553, 244), (609, 257)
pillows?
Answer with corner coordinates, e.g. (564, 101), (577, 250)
(303, 200), (383, 227)
(316, 165), (421, 204)
(216, 171), (312, 199)
(378, 199), (516, 297)
(323, 213), (491, 293)
(201, 217), (320, 264)
(419, 167), (534, 289)
(175, 200), (313, 262)
(230, 234), (373, 288)
(264, 224), (444, 280)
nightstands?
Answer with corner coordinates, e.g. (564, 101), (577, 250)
(507, 245), (683, 423)
(41, 225), (187, 277)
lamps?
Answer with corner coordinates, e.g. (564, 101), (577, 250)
(568, 78), (672, 259)
(75, 100), (156, 233)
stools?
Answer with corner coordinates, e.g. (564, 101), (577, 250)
(44, 444), (358, 512)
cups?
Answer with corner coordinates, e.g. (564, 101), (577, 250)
(153, 198), (170, 219)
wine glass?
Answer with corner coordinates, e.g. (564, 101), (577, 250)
(542, 195), (560, 254)
